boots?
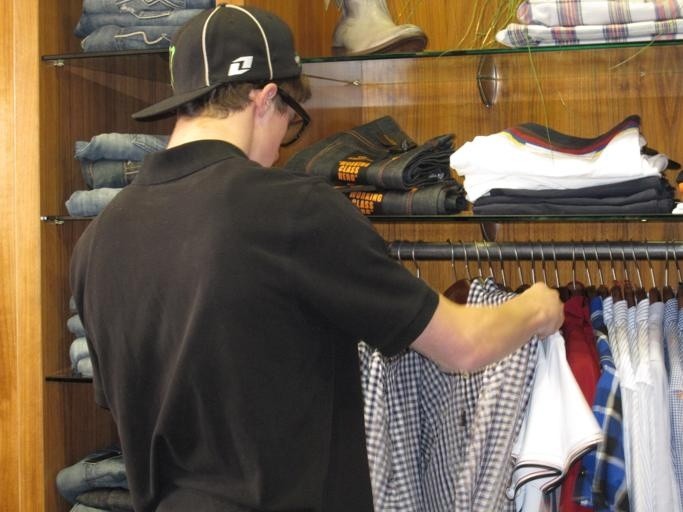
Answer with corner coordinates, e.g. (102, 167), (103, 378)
(331, 0), (427, 56)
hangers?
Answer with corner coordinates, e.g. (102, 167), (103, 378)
(388, 239), (683, 309)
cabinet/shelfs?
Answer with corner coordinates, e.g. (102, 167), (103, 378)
(42, 50), (170, 383)
(299, 41), (683, 222)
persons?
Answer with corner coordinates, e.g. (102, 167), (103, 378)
(69, 2), (564, 512)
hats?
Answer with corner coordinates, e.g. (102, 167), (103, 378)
(131, 3), (303, 122)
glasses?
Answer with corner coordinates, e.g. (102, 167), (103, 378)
(256, 83), (309, 147)
